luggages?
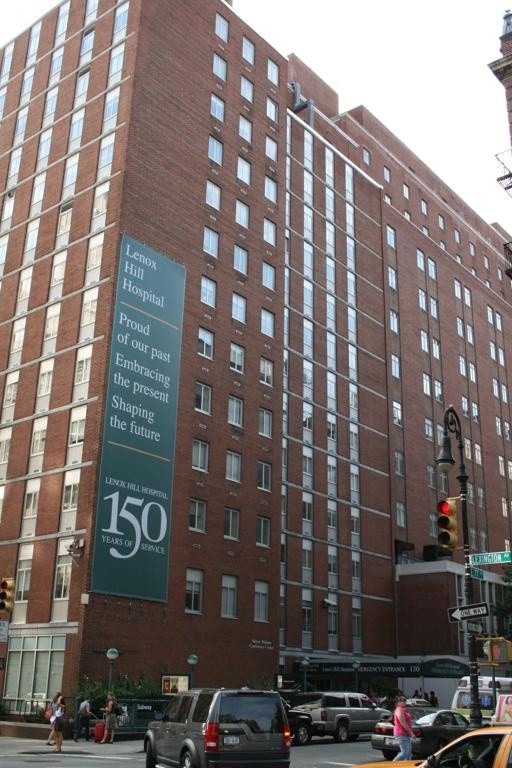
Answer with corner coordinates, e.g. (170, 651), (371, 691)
(95, 713), (107, 741)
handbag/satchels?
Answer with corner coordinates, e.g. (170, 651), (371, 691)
(114, 702), (123, 716)
(80, 709), (91, 718)
(45, 703), (52, 720)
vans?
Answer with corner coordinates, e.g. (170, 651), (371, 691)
(451, 676), (512, 718)
(143, 687), (292, 768)
(408, 698), (431, 706)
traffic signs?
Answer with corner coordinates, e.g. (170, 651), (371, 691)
(471, 567), (484, 581)
(469, 550), (512, 566)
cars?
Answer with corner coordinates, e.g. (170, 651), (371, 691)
(371, 706), (481, 759)
(351, 726), (512, 768)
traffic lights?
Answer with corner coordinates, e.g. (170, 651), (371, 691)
(0, 578), (14, 611)
(437, 498), (459, 549)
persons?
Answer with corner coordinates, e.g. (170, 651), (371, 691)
(421, 693), (428, 700)
(46, 692), (62, 745)
(413, 690), (421, 698)
(392, 695), (416, 761)
(99, 691), (119, 744)
(429, 690), (439, 707)
(376, 685), (389, 703)
(502, 696), (512, 721)
(389, 688), (402, 703)
(164, 680), (170, 692)
(74, 696), (92, 742)
(54, 695), (67, 753)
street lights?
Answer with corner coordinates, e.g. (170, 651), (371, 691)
(447, 602), (489, 624)
(437, 405), (483, 758)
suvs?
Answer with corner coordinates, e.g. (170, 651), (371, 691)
(290, 690), (393, 742)
(281, 697), (313, 745)
